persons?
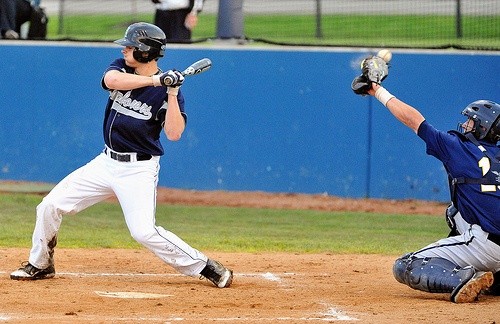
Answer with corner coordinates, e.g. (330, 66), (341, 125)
(152, 0), (205, 43)
(361, 56), (500, 303)
(0, 0), (46, 39)
(11, 22), (233, 288)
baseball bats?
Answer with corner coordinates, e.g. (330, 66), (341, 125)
(164, 57), (212, 87)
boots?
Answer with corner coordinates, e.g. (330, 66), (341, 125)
(451, 266), (494, 303)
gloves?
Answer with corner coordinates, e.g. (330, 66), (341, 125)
(166, 69), (185, 95)
(153, 70), (178, 88)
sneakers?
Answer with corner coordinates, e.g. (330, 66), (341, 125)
(10, 261), (55, 280)
(199, 259), (233, 288)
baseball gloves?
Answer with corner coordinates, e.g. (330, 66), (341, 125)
(351, 55), (389, 95)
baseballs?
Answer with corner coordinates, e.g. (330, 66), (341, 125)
(377, 48), (393, 64)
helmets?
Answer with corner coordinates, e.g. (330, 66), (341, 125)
(457, 100), (500, 145)
(113, 22), (166, 64)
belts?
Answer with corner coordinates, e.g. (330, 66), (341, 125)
(104, 148), (152, 162)
(487, 233), (500, 246)
